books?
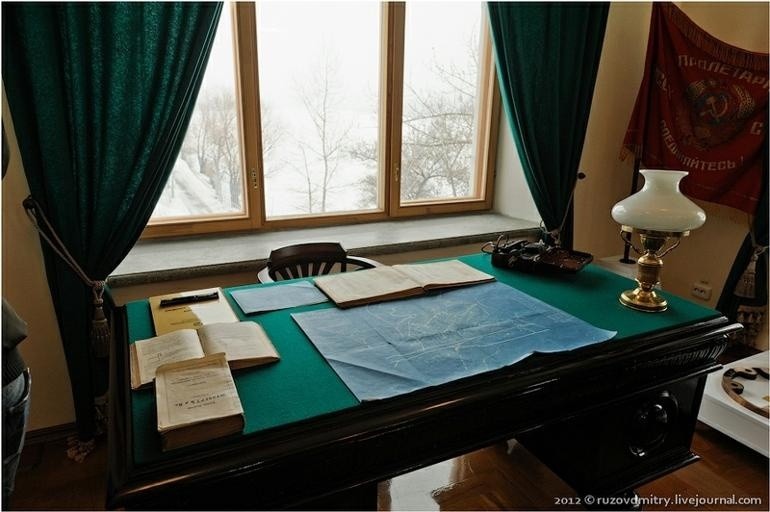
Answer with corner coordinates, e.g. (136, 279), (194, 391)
(154, 352), (248, 451)
(129, 321), (282, 390)
(148, 286), (242, 338)
(314, 259), (498, 310)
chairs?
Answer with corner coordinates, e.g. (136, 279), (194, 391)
(258, 244), (391, 286)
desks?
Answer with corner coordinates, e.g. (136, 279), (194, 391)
(106, 243), (747, 509)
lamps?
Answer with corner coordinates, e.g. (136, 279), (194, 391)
(613, 160), (709, 312)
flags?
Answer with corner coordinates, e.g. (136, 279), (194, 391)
(617, 2), (769, 229)
